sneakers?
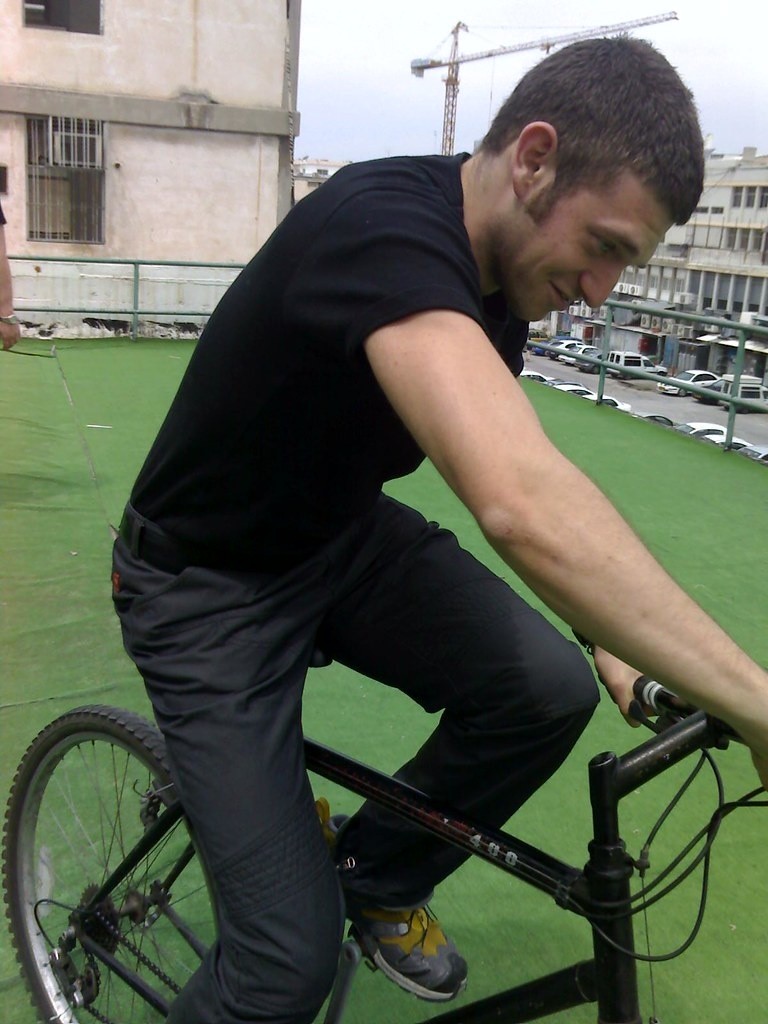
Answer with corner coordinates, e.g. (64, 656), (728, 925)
(316, 797), (468, 1002)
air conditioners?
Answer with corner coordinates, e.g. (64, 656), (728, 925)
(567, 300), (608, 320)
(48, 130), (104, 168)
(615, 282), (642, 294)
(648, 287), (692, 305)
(640, 313), (693, 340)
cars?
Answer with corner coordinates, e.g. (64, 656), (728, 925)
(574, 350), (640, 374)
(534, 336), (583, 356)
(545, 339), (586, 360)
(519, 366), (767, 467)
(558, 345), (601, 366)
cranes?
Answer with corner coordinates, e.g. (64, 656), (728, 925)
(410, 13), (683, 158)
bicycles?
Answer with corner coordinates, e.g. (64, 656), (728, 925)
(0, 624), (768, 1024)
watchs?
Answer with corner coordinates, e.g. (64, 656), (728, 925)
(0, 315), (17, 325)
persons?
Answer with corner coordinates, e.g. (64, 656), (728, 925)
(526, 337), (533, 362)
(112, 33), (767, 1024)
(0, 207), (22, 351)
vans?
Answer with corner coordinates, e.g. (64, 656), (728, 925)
(607, 350), (668, 381)
